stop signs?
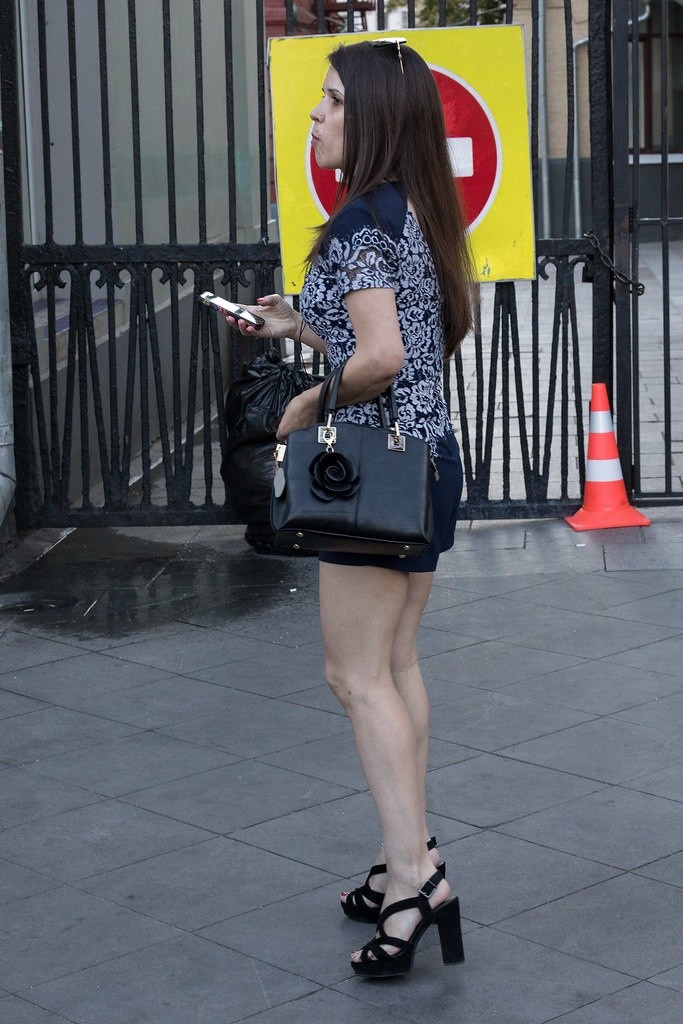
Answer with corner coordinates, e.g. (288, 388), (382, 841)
(306, 64), (502, 242)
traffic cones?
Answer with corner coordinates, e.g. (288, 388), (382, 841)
(563, 383), (652, 532)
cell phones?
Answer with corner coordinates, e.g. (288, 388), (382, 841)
(198, 292), (265, 329)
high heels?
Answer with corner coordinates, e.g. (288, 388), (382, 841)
(350, 870), (464, 978)
(341, 834), (445, 924)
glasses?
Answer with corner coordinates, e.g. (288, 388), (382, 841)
(373, 37), (407, 77)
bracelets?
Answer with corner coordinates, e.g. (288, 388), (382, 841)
(299, 320), (306, 350)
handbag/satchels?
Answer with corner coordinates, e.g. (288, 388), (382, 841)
(266, 359), (441, 559)
(218, 336), (326, 516)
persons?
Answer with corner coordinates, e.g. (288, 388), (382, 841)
(201, 41), (464, 977)
